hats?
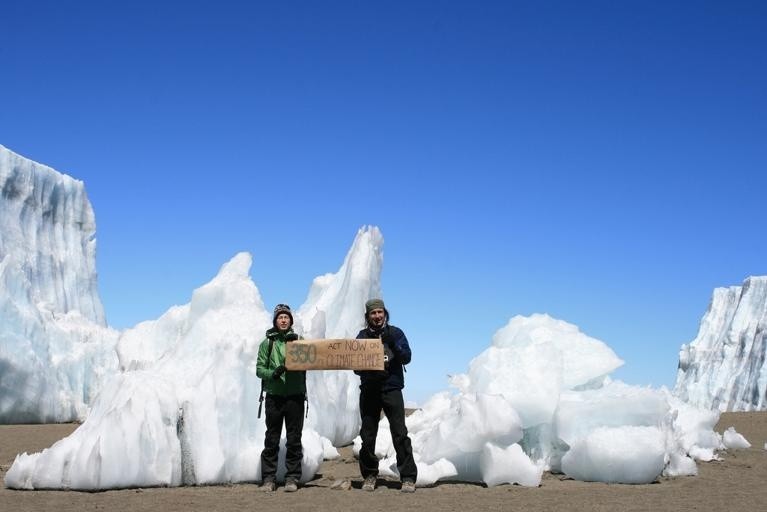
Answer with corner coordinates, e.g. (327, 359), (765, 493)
(365, 299), (388, 317)
(273, 303), (294, 327)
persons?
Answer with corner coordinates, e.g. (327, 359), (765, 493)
(256, 303), (306, 492)
(353, 299), (417, 493)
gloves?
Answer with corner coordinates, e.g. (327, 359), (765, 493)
(381, 332), (394, 344)
(272, 366), (288, 379)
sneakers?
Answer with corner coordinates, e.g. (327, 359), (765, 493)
(362, 473), (378, 491)
(284, 478), (298, 493)
(402, 478), (416, 493)
(258, 480), (276, 492)
